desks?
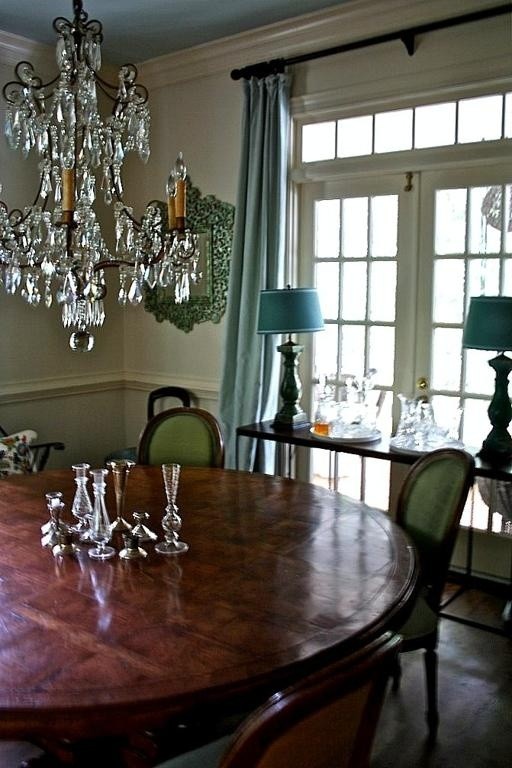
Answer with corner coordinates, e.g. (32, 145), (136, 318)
(3, 465), (422, 768)
(234, 411), (512, 646)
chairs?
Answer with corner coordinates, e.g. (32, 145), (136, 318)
(136, 383), (227, 476)
(386, 448), (475, 734)
(219, 632), (406, 764)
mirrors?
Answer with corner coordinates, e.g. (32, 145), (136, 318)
(131, 171), (234, 336)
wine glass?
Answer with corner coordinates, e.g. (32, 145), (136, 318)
(88, 468), (115, 558)
(154, 463), (190, 554)
(70, 463), (93, 533)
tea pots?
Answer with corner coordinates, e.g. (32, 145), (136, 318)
(395, 391), (449, 449)
(311, 368), (383, 438)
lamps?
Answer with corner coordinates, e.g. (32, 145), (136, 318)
(2, 0), (194, 352)
(457, 296), (512, 465)
(251, 285), (326, 432)
